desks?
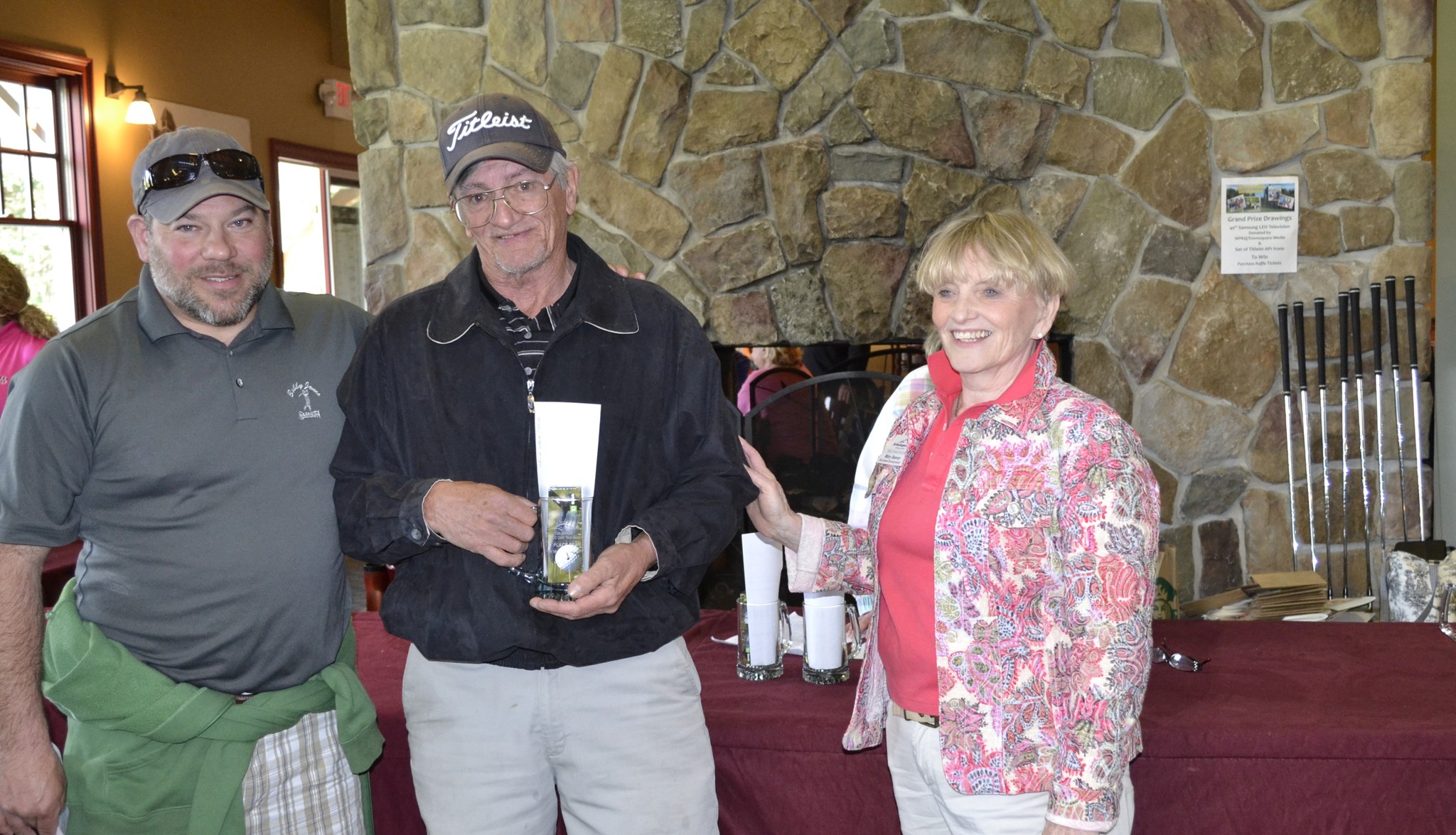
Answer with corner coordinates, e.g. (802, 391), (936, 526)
(40, 607), (1456, 835)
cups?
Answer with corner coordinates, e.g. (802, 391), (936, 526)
(507, 497), (592, 603)
(1439, 583), (1456, 641)
(737, 595), (794, 682)
(801, 601), (863, 684)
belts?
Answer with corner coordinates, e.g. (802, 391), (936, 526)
(893, 702), (939, 728)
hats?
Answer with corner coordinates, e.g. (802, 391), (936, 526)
(438, 91), (566, 196)
(131, 126), (270, 224)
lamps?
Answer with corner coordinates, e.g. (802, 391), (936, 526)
(105, 73), (157, 126)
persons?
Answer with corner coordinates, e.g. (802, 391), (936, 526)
(736, 210), (1161, 835)
(328, 93), (760, 835)
(1, 126), (375, 834)
(0, 249), (83, 607)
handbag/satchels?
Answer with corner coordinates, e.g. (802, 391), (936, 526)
(1394, 539), (1447, 563)
(1380, 547), (1456, 623)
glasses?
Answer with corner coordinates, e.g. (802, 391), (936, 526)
(449, 171), (555, 229)
(136, 149), (265, 215)
(1151, 642), (1211, 672)
